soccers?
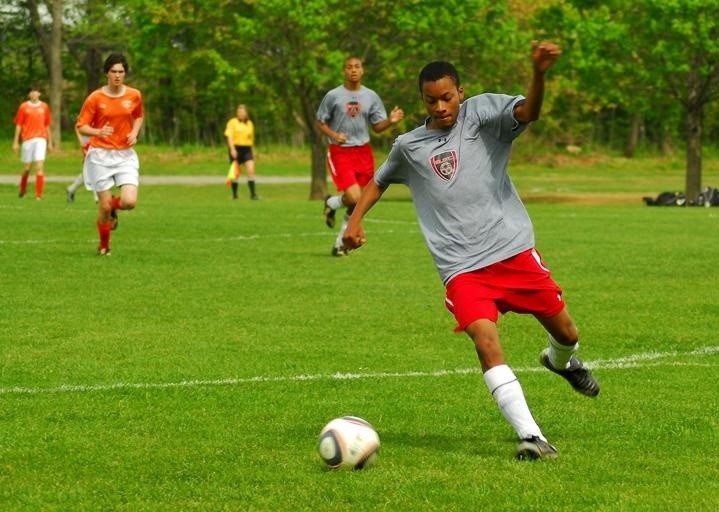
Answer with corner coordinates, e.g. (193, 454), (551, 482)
(318, 416), (380, 470)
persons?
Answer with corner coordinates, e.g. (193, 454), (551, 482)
(316, 56), (404, 256)
(66, 54), (145, 256)
(11, 89), (53, 200)
(224, 104), (260, 201)
(342, 40), (601, 459)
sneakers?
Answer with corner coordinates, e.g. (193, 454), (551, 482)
(97, 248), (112, 257)
(540, 347), (600, 397)
(111, 210), (120, 231)
(332, 247), (348, 257)
(515, 436), (558, 460)
(66, 187), (75, 201)
(323, 194), (335, 228)
